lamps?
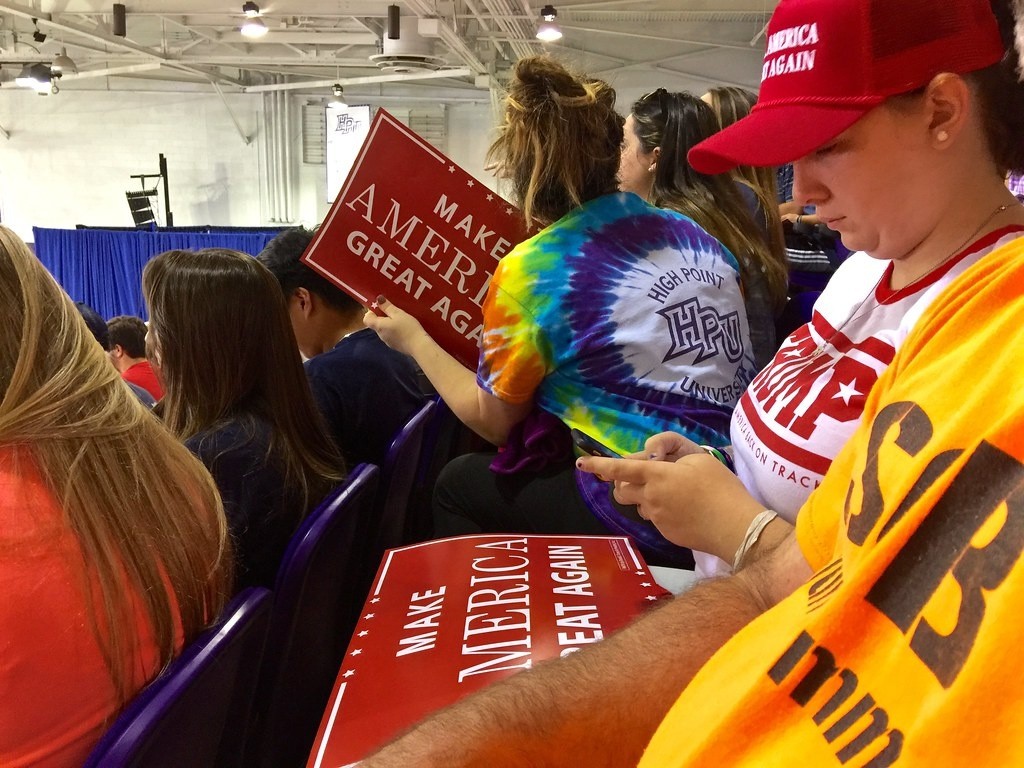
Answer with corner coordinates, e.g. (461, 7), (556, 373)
(50, 30), (78, 75)
(15, 62), (51, 92)
(32, 18), (47, 43)
(535, 0), (563, 42)
(328, 65), (348, 108)
(113, 0), (126, 37)
(388, 4), (400, 40)
(241, 0), (267, 38)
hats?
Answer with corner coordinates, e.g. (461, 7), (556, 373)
(687, 0), (1005, 176)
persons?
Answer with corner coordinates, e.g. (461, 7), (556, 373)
(140, 247), (348, 599)
(106, 314), (168, 403)
(0, 225), (229, 768)
(700, 86), (787, 266)
(254, 224), (425, 487)
(575, 0), (1024, 603)
(363, 54), (760, 571)
(354, 1), (1023, 768)
(75, 301), (158, 411)
(614, 89), (792, 373)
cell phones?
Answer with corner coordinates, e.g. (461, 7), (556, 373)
(569, 427), (624, 461)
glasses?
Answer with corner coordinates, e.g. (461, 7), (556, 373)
(641, 88), (669, 124)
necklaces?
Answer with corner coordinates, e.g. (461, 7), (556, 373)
(811, 200), (1020, 358)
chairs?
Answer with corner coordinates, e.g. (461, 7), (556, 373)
(89, 396), (490, 768)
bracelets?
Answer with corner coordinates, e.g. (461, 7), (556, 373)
(732, 510), (777, 574)
(699, 445), (736, 474)
(795, 215), (802, 224)
